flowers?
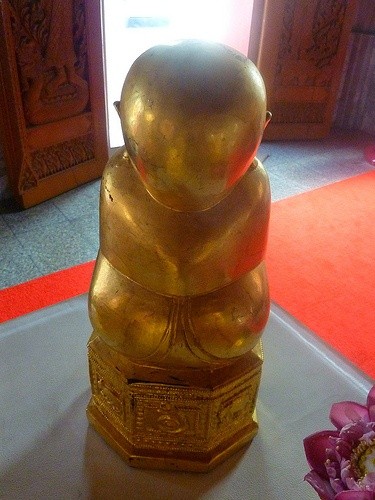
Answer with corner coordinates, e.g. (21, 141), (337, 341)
(302, 384), (375, 500)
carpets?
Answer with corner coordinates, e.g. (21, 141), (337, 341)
(265, 168), (375, 380)
